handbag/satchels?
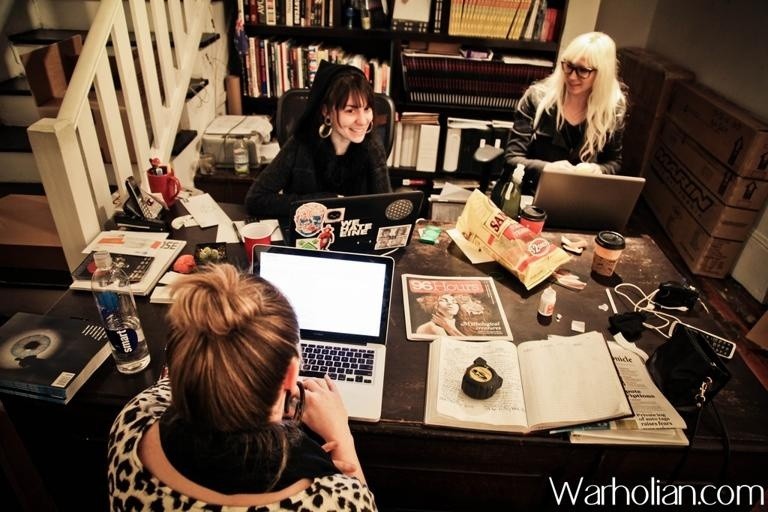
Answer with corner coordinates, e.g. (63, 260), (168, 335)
(645, 323), (732, 414)
(491, 164), (525, 221)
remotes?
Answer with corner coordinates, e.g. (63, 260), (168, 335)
(668, 321), (737, 359)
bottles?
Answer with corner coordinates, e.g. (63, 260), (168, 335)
(232, 134), (250, 177)
(537, 288), (557, 317)
(89, 249), (152, 375)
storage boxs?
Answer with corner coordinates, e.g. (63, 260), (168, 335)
(617, 47), (766, 280)
(20, 33), (143, 163)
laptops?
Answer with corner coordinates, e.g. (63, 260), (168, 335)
(289, 189), (425, 253)
(251, 243), (394, 423)
(531, 166), (647, 231)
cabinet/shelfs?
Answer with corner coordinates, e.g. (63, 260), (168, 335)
(225, 1), (570, 180)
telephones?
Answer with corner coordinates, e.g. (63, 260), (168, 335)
(115, 175), (176, 238)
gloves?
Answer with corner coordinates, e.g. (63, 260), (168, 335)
(543, 160), (602, 177)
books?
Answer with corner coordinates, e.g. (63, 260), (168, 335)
(0, 386), (70, 407)
(400, 270), (515, 342)
(240, 0), (558, 178)
(565, 337), (690, 449)
(68, 233), (189, 299)
(0, 311), (112, 400)
(420, 325), (633, 437)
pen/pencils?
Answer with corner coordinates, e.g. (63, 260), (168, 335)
(149, 158), (157, 175)
(605, 288), (618, 315)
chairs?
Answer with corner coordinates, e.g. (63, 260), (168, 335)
(276, 89), (396, 163)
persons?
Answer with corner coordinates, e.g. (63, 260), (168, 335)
(244, 57), (393, 237)
(490, 31), (635, 212)
(105, 259), (379, 512)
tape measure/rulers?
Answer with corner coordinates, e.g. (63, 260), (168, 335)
(462, 356), (503, 399)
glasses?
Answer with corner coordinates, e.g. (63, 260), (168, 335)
(561, 61), (596, 79)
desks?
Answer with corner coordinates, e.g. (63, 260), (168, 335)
(0, 201), (767, 511)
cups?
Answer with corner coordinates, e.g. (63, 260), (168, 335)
(243, 222), (272, 265)
(147, 165), (182, 207)
(199, 152), (216, 176)
(591, 230), (626, 278)
(520, 206), (548, 236)
